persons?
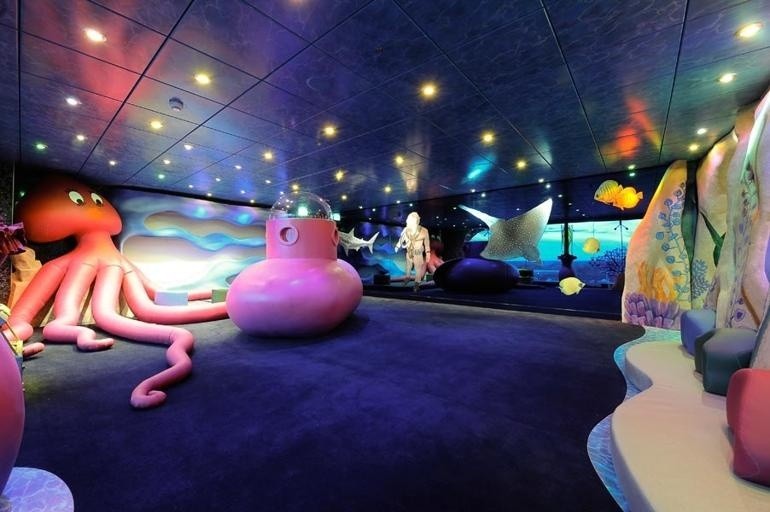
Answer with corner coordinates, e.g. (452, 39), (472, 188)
(393, 210), (431, 292)
